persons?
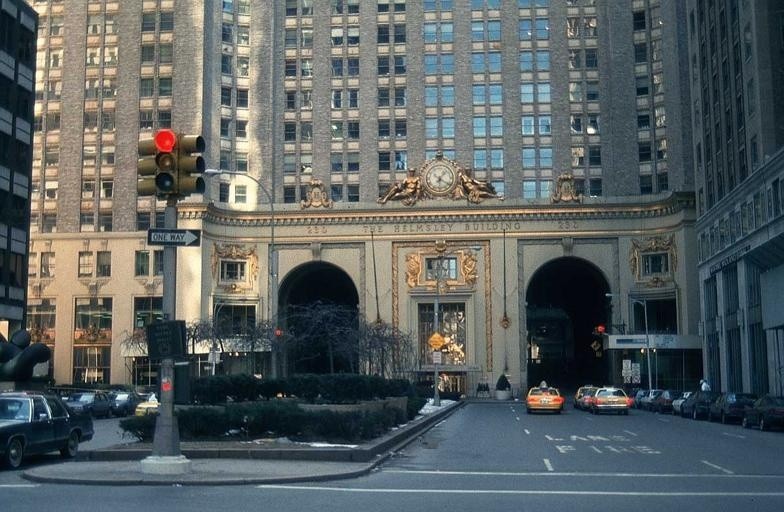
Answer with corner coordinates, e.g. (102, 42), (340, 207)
(459, 249), (478, 284)
(375, 168), (421, 204)
(458, 166), (504, 204)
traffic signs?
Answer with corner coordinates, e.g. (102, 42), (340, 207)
(148, 229), (200, 247)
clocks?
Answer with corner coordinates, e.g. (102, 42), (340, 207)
(420, 161), (457, 196)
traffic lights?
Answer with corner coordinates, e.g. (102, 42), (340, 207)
(138, 130), (206, 196)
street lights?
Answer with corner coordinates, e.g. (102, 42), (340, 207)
(207, 168), (277, 377)
(606, 293), (652, 388)
(433, 246), (482, 407)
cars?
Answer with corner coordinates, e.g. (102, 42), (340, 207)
(575, 386), (632, 416)
(635, 389), (784, 432)
(526, 381), (565, 415)
(0, 389), (158, 467)
